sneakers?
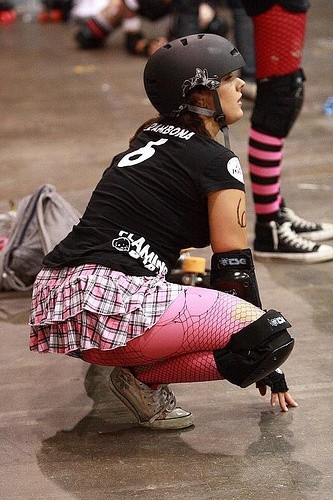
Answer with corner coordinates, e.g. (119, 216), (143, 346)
(279, 205), (333, 241)
(108, 365), (195, 430)
(83, 364), (127, 414)
(253, 221), (333, 263)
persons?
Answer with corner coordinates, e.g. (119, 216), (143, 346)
(242, 0), (333, 264)
(28, 33), (298, 431)
(0, 0), (257, 100)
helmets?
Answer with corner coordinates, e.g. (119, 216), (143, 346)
(143, 34), (246, 118)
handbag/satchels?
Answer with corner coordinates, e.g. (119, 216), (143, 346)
(0, 184), (83, 292)
